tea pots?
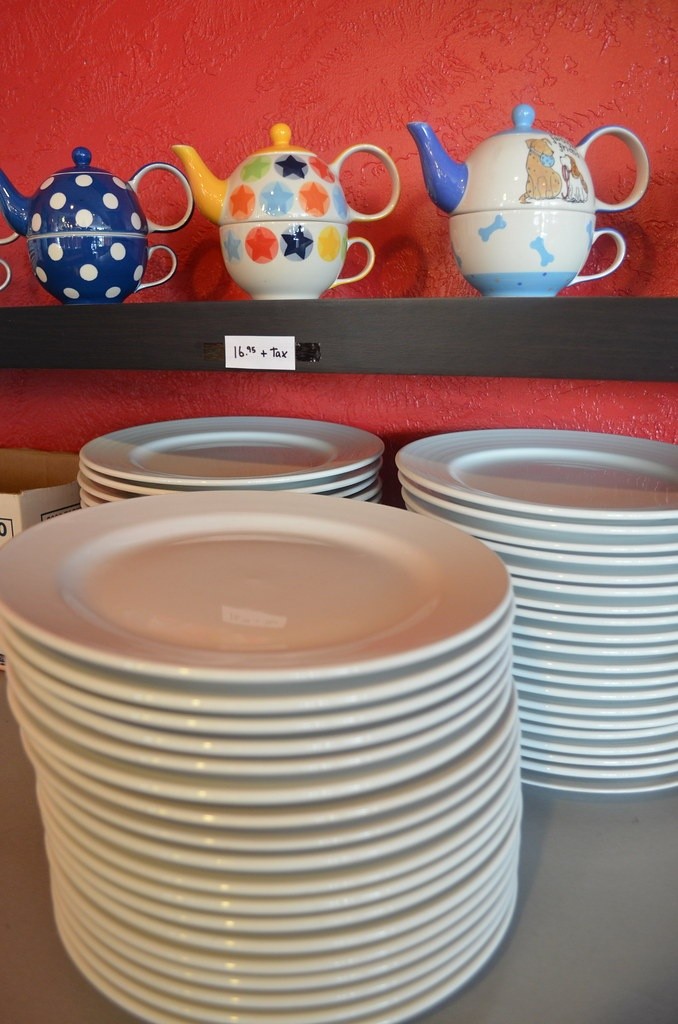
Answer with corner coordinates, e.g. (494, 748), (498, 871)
(170, 125), (398, 300)
(404, 99), (652, 300)
(1, 141), (194, 303)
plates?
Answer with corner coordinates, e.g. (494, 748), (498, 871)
(0, 412), (678, 1024)
